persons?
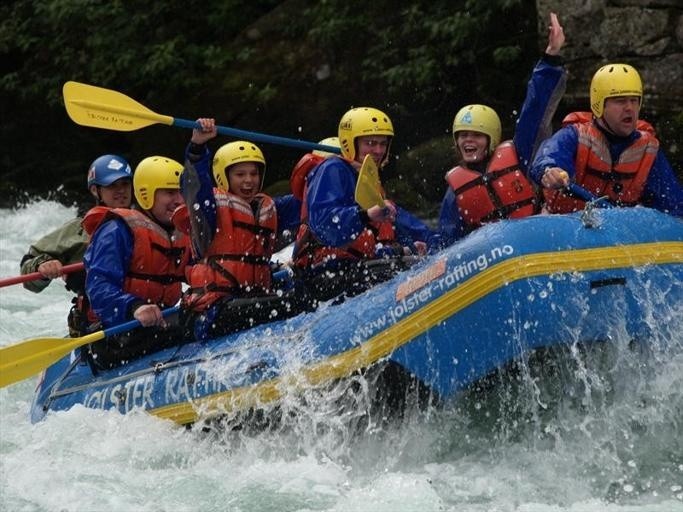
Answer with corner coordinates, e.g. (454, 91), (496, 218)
(83, 156), (205, 360)
(305, 135), (341, 158)
(21, 153), (133, 338)
(180, 118), (303, 341)
(293, 106), (436, 300)
(528, 63), (683, 217)
(437, 12), (566, 258)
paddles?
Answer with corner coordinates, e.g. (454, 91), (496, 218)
(62, 81), (344, 157)
(354, 154), (430, 239)
(0, 305), (183, 387)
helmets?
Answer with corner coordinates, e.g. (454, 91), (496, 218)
(338, 107), (395, 167)
(87, 155), (133, 189)
(133, 154), (185, 210)
(452, 104), (502, 156)
(312, 137), (340, 157)
(590, 63), (644, 119)
(212, 140), (266, 192)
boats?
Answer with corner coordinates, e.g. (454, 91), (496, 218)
(28, 206), (683, 447)
